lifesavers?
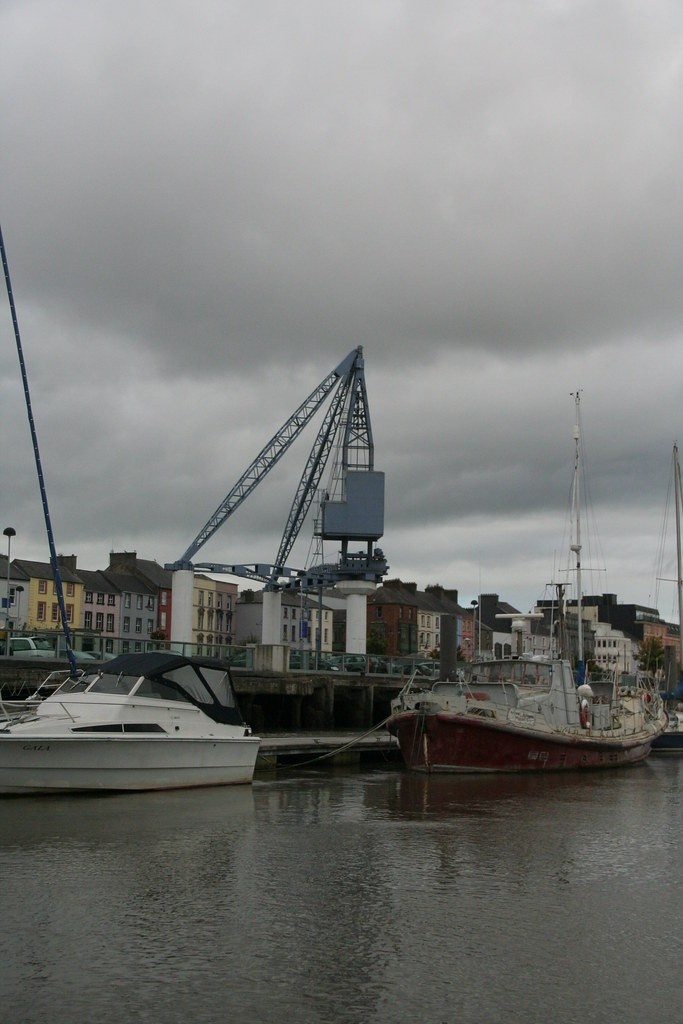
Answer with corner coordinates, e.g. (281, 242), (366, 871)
(582, 699), (590, 726)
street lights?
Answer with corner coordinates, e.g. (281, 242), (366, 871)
(3, 527), (16, 655)
(570, 544), (584, 686)
(296, 570), (307, 650)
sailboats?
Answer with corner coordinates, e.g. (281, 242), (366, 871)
(637, 441), (683, 752)
(386, 387), (668, 777)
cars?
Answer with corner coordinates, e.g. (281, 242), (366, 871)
(328, 654), (440, 676)
(10, 637), (56, 658)
(289, 655), (347, 672)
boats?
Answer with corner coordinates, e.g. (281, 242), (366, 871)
(0, 653), (263, 789)
